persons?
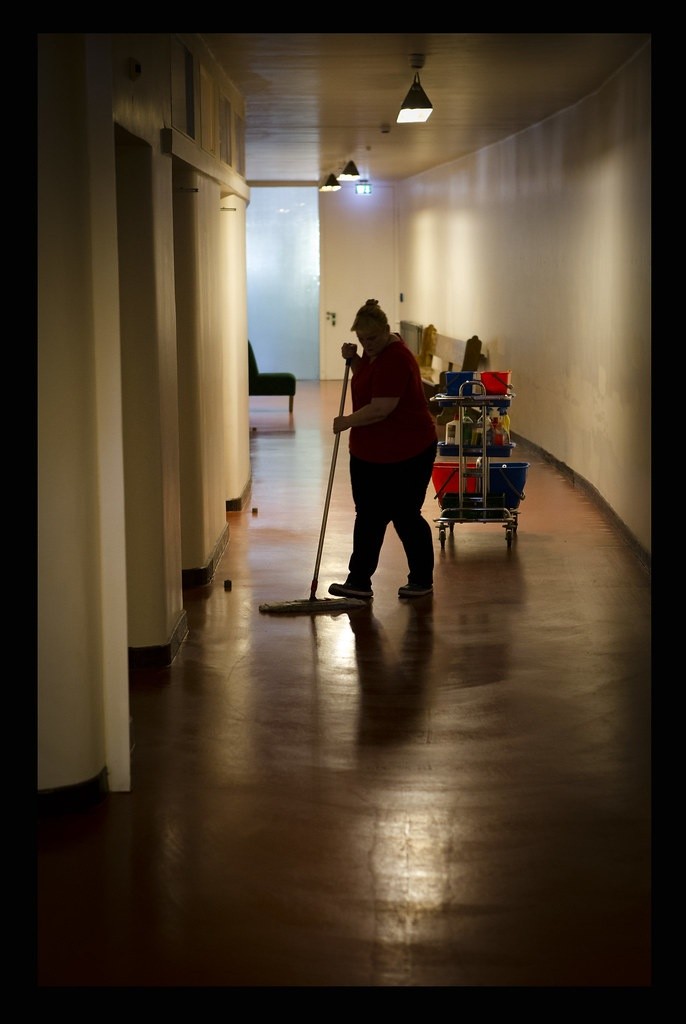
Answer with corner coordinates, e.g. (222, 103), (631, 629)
(328, 299), (438, 595)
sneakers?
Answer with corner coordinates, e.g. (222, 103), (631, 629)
(328, 580), (373, 598)
(398, 584), (433, 596)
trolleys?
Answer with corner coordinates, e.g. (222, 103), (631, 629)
(426, 381), (521, 549)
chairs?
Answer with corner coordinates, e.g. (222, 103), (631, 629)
(248, 341), (295, 412)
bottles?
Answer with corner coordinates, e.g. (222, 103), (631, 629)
(446, 406), (510, 445)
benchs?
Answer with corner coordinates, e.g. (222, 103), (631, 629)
(413, 323), (486, 393)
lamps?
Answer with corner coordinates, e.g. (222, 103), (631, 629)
(337, 161), (360, 182)
(396, 74), (433, 122)
(318, 173), (341, 192)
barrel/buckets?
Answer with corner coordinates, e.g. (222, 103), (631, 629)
(431, 462), (477, 511)
(480, 371), (514, 395)
(479, 462), (531, 509)
(445, 371), (478, 396)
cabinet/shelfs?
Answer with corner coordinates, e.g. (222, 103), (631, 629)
(433, 382), (521, 544)
